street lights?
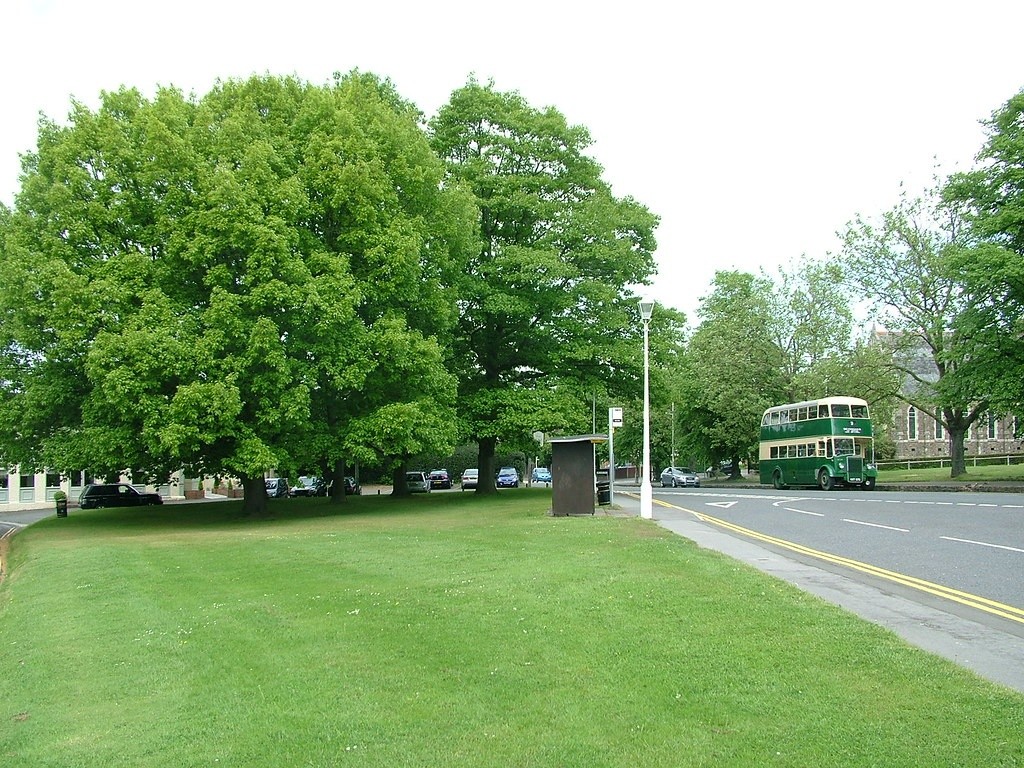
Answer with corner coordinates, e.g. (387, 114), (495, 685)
(636, 297), (656, 519)
(535, 456), (540, 468)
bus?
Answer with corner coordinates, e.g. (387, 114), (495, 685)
(757, 395), (877, 492)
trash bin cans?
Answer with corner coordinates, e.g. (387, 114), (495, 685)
(597, 480), (610, 505)
(56, 498), (68, 518)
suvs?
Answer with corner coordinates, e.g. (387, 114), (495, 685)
(496, 467), (519, 488)
(427, 469), (454, 489)
(77, 482), (164, 510)
(406, 471), (431, 493)
(460, 468), (478, 489)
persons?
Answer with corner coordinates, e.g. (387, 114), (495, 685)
(784, 448), (842, 457)
(789, 407), (865, 421)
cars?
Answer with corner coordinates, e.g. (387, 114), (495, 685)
(531, 468), (552, 483)
(327, 476), (363, 497)
(660, 467), (701, 488)
(706, 461), (741, 477)
(264, 477), (288, 498)
(289, 475), (327, 498)
